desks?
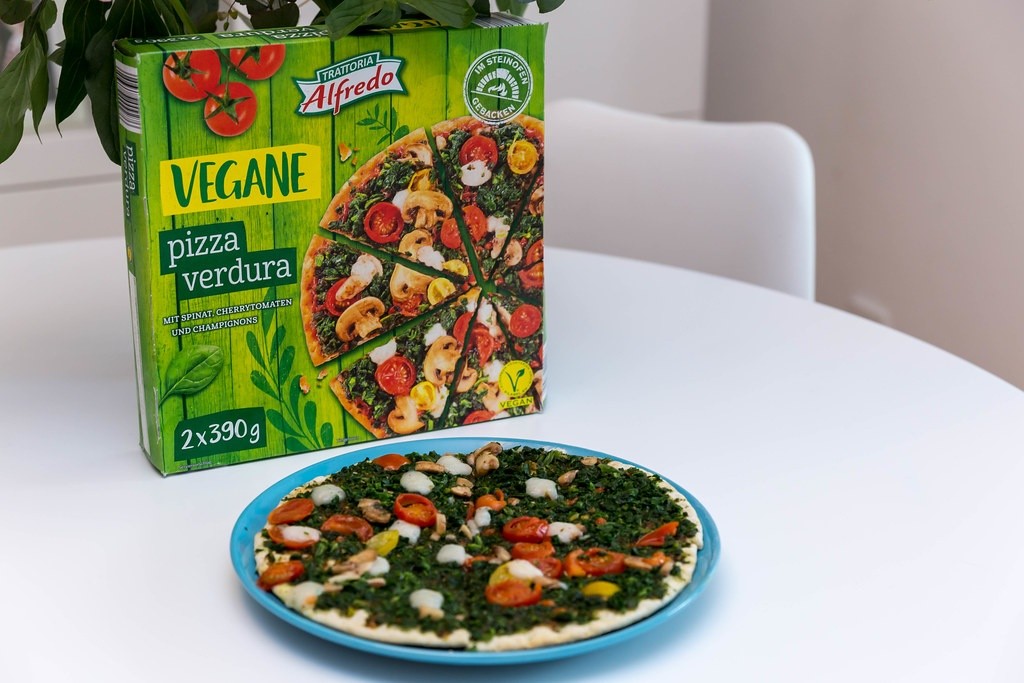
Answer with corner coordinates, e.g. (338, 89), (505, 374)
(0, 235), (1024, 683)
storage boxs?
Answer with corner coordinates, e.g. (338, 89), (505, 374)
(113, 15), (546, 473)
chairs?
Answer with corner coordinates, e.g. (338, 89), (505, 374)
(547, 98), (816, 299)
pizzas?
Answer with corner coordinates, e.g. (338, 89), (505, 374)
(300, 112), (544, 440)
(254, 441), (703, 651)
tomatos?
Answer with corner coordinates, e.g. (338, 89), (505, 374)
(162, 42), (285, 136)
